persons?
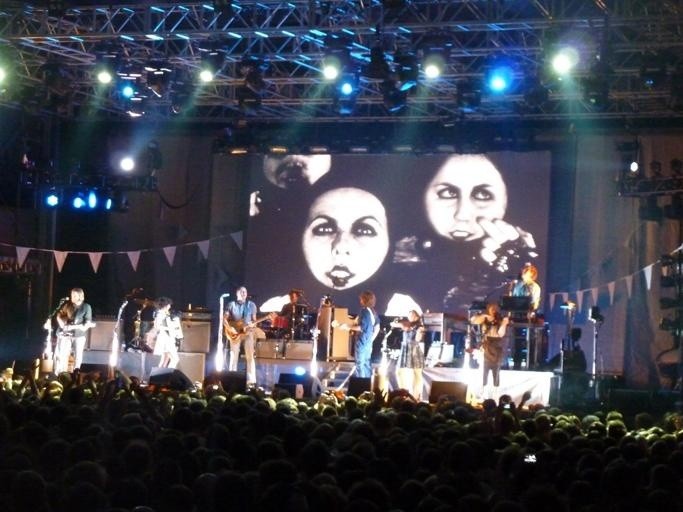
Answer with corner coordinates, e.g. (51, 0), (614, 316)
(249, 154), (333, 223)
(505, 264), (541, 368)
(255, 168), (425, 318)
(1, 288), (682, 511)
(394, 149), (543, 313)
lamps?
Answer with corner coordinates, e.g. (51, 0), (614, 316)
(658, 249), (683, 337)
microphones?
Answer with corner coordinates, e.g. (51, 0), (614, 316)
(502, 278), (518, 286)
(290, 288), (303, 295)
(61, 296), (70, 301)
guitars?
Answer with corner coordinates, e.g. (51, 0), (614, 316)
(331, 315), (359, 336)
(224, 312), (278, 344)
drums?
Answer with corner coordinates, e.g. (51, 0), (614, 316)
(262, 320), (271, 332)
(301, 315), (313, 326)
(270, 316), (288, 329)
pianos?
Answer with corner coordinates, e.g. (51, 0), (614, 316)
(420, 312), (465, 363)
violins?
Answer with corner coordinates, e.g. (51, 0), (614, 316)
(401, 320), (420, 338)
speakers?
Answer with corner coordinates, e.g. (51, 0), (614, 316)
(279, 373), (322, 398)
(149, 367), (195, 392)
